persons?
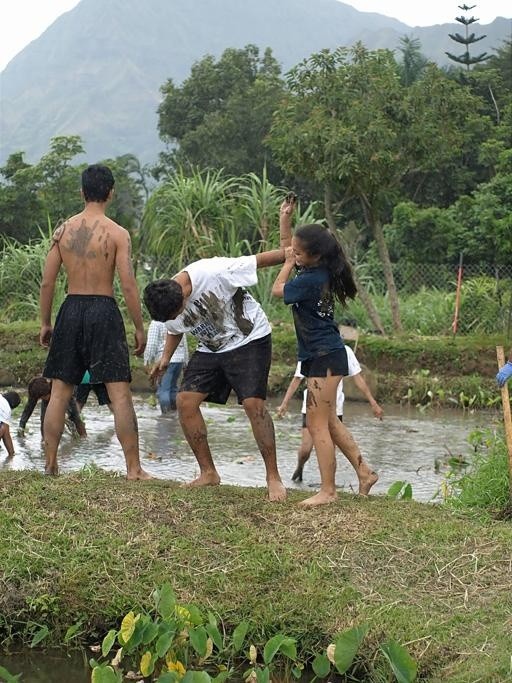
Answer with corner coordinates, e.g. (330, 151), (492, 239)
(0, 390), (20, 456)
(19, 319), (116, 442)
(38, 161), (155, 480)
(276, 344), (382, 483)
(268, 195), (380, 509)
(143, 247), (300, 506)
(141, 319), (189, 415)
(494, 349), (512, 388)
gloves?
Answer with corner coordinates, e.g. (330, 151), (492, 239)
(496, 361), (512, 388)
